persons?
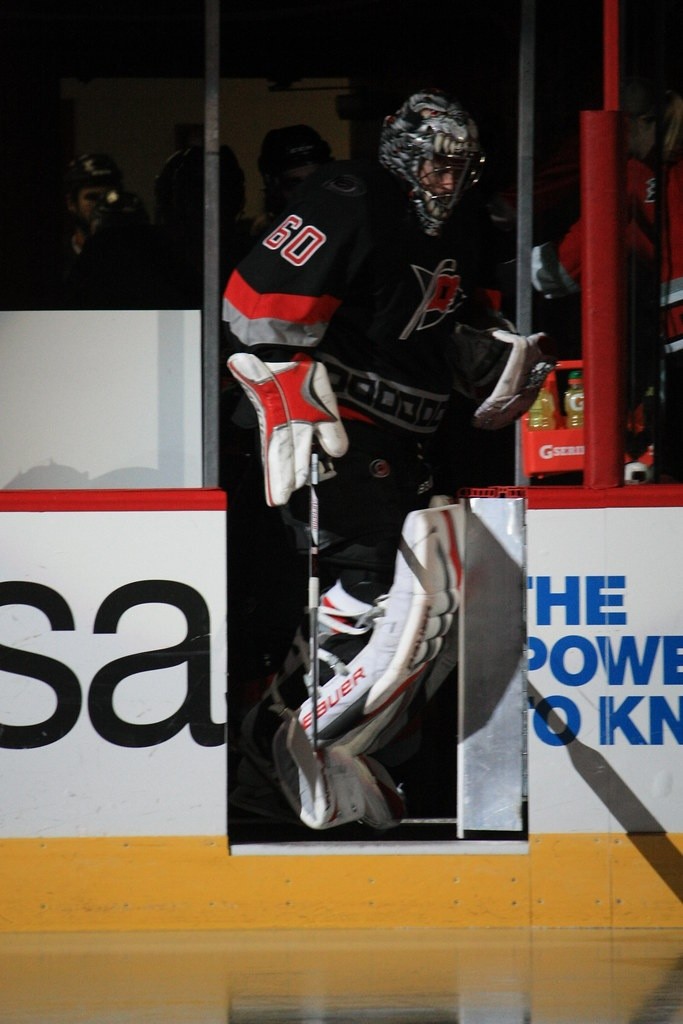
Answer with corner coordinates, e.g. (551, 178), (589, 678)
(46, 70), (683, 581)
(222, 94), (552, 841)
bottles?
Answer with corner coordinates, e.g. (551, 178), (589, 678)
(529, 385), (555, 430)
(565, 371), (583, 429)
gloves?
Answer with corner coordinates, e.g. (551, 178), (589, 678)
(553, 158), (657, 288)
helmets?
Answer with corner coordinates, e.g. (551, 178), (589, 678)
(154, 145), (245, 231)
(258, 125), (333, 187)
(379, 88), (486, 236)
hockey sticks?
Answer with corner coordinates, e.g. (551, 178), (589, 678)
(285, 444), (331, 798)
